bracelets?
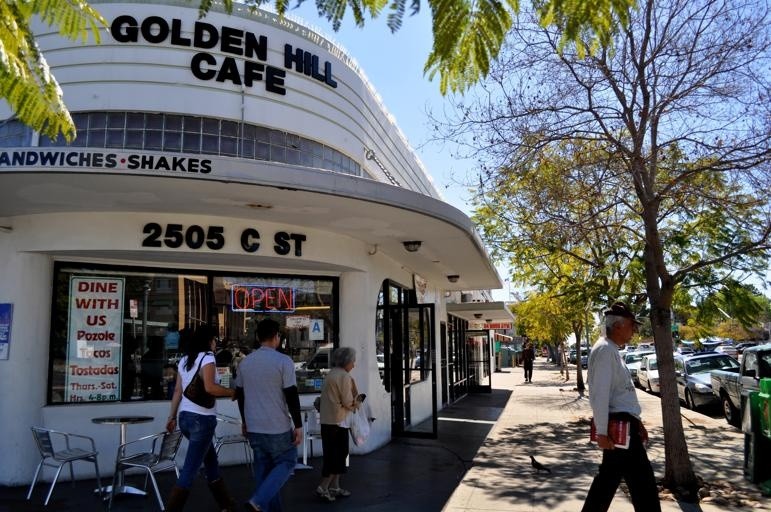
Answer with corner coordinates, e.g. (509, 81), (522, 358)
(169, 416), (178, 419)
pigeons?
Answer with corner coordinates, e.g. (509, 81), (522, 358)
(529, 455), (551, 474)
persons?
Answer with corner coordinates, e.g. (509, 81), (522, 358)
(230, 317), (303, 511)
(164, 323), (243, 512)
(580, 302), (662, 512)
(314, 346), (362, 503)
(122, 327), (250, 401)
(523, 342), (536, 383)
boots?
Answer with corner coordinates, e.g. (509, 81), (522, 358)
(165, 485), (190, 512)
(208, 478), (241, 512)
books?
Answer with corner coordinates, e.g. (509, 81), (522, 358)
(591, 417), (632, 450)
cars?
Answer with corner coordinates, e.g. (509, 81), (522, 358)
(565, 334), (758, 411)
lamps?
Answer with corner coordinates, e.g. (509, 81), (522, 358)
(447, 275), (460, 283)
(403, 240), (422, 253)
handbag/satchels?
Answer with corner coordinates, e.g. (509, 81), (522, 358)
(183, 373), (215, 409)
(313, 398), (320, 412)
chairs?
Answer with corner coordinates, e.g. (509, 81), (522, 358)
(212, 409), (255, 479)
(26, 425), (184, 512)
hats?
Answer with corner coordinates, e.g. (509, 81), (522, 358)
(604, 302), (644, 324)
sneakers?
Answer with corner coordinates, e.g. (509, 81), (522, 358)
(245, 500), (261, 512)
(313, 486), (335, 502)
(329, 488), (351, 497)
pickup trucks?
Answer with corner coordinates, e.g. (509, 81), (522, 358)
(710, 343), (771, 425)
